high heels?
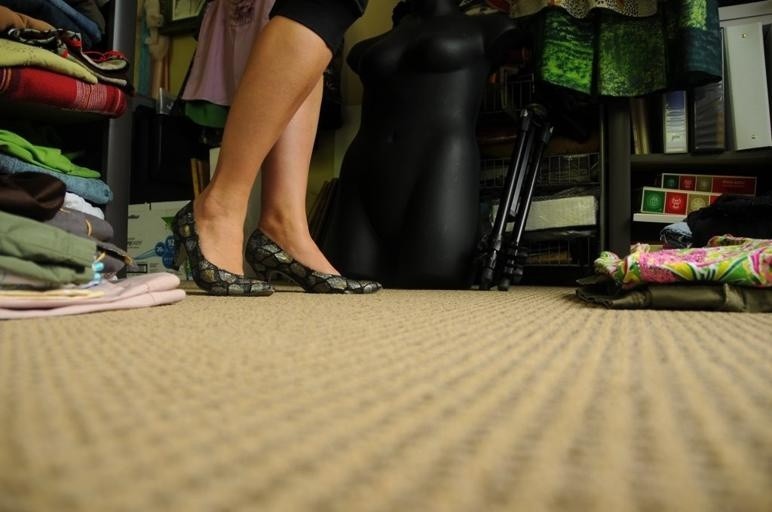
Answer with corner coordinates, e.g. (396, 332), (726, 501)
(245, 227), (381, 293)
(171, 200), (273, 296)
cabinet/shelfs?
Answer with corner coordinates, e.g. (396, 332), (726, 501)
(463, 1), (771, 289)
(0, 1), (141, 285)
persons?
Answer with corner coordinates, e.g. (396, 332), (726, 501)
(168, 0), (384, 298)
